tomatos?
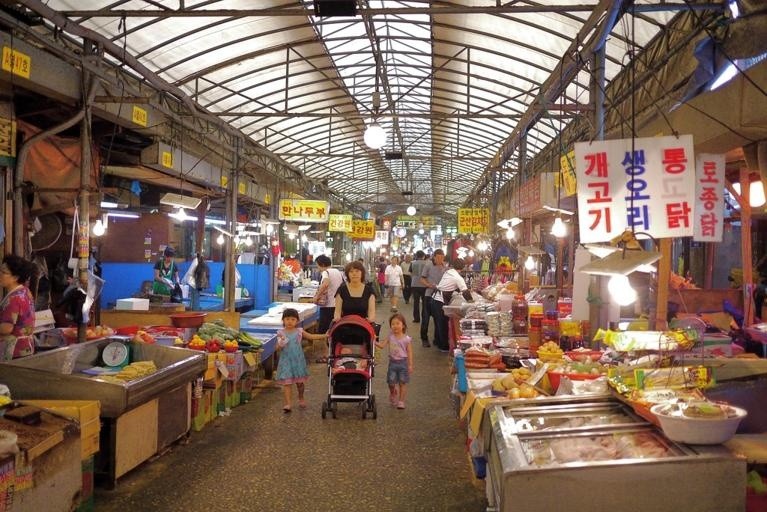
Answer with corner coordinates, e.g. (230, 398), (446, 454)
(204, 338), (221, 351)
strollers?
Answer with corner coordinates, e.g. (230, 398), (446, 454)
(316, 314), (379, 420)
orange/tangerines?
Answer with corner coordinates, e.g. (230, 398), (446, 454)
(223, 339), (238, 347)
(189, 334), (206, 346)
(508, 387), (534, 398)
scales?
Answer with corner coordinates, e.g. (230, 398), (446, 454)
(238, 285), (250, 298)
(102, 335), (130, 370)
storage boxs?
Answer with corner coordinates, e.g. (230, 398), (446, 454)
(192, 349), (253, 432)
(16, 397), (103, 512)
(448, 332), (494, 486)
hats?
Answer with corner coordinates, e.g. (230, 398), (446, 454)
(165, 247), (176, 256)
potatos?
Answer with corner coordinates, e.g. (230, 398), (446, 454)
(512, 368), (530, 380)
(537, 340), (563, 354)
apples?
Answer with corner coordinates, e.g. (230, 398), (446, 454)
(87, 324), (114, 339)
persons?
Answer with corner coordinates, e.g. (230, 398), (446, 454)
(333, 347), (367, 373)
(0, 254), (40, 360)
(153, 246), (181, 296)
(308, 247), (475, 352)
(276, 306), (332, 412)
(373, 312), (416, 413)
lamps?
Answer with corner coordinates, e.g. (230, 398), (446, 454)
(505, 208), (659, 308)
(406, 197), (417, 215)
(364, 91), (387, 152)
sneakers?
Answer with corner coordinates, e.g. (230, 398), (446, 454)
(440, 346), (449, 352)
(423, 340), (430, 347)
(390, 389), (397, 400)
(390, 308), (398, 312)
(298, 400), (307, 409)
(283, 404), (292, 411)
(397, 401), (405, 409)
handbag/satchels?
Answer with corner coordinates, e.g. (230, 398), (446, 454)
(313, 288), (328, 306)
